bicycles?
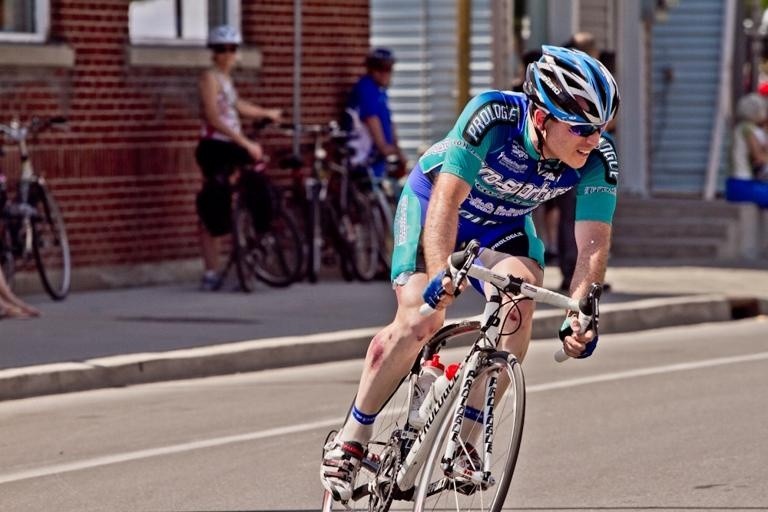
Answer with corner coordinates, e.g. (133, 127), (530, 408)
(1, 112), (79, 298)
(314, 236), (607, 512)
(224, 106), (396, 288)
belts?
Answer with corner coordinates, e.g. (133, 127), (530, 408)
(200, 128), (232, 142)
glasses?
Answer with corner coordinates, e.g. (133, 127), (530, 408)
(567, 125), (601, 137)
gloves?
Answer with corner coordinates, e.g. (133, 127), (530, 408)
(424, 266), (466, 311)
(557, 313), (599, 360)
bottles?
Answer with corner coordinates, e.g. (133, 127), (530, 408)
(418, 362), (461, 422)
(407, 353), (445, 426)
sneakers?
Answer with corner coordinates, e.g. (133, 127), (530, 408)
(451, 441), (497, 493)
(543, 251), (561, 266)
(199, 270), (246, 293)
(319, 426), (370, 502)
(560, 280), (611, 297)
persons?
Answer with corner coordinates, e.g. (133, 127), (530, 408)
(512, 50), (560, 267)
(0, 267), (43, 321)
(558, 31), (616, 296)
(193, 25), (286, 294)
(319, 44), (622, 503)
(724, 92), (768, 208)
(337, 49), (406, 194)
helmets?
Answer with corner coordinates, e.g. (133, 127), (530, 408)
(207, 24), (242, 49)
(521, 43), (622, 127)
(365, 47), (394, 67)
